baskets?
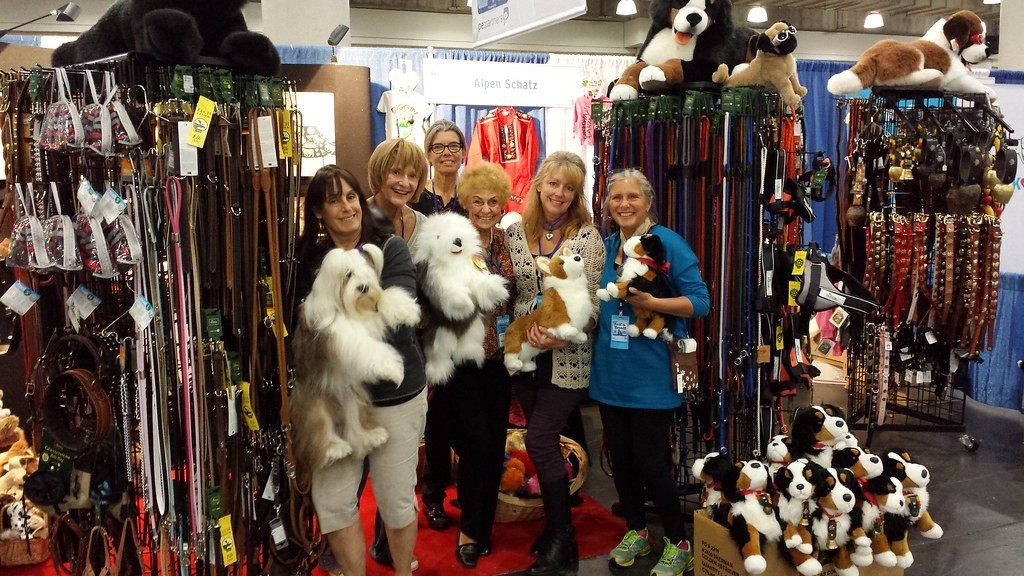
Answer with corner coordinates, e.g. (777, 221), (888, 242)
(493, 429), (588, 522)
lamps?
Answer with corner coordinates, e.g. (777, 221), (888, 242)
(0, 2), (82, 38)
(328, 25), (350, 63)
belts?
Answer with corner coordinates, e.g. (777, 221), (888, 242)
(135, 99), (307, 575)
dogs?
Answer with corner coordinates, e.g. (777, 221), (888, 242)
(411, 213), (510, 385)
(283, 242), (422, 472)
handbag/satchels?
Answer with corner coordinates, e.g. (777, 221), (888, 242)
(668, 338), (699, 390)
(7, 66), (143, 280)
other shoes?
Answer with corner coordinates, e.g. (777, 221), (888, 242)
(319, 554), (345, 576)
(372, 546), (419, 572)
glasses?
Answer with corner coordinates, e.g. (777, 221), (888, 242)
(430, 142), (463, 154)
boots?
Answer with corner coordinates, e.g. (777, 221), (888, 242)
(527, 525), (579, 576)
(530, 524), (567, 557)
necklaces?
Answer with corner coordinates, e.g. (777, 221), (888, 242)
(541, 213), (569, 240)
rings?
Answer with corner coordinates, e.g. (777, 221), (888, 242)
(624, 300), (627, 302)
(533, 343), (536, 346)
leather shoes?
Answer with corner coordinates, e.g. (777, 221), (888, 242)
(423, 495), (448, 530)
(477, 535), (491, 556)
(455, 531), (478, 568)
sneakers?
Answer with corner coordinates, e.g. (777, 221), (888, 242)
(607, 529), (649, 567)
(651, 536), (693, 576)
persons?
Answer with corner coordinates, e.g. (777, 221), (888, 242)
(587, 170), (709, 576)
(280, 163), (430, 576)
(422, 162), (516, 568)
(312, 137), (430, 576)
(409, 119), (470, 219)
(505, 150), (608, 576)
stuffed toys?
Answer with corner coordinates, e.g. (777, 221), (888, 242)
(692, 403), (944, 576)
(502, 245), (594, 376)
(283, 243), (421, 470)
(50, 0), (281, 77)
(609, 0), (808, 105)
(596, 234), (681, 342)
(826, 10), (998, 102)
(409, 211), (510, 384)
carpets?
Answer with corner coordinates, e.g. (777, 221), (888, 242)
(1, 445), (628, 576)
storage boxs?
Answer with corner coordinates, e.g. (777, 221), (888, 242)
(693, 509), (903, 576)
